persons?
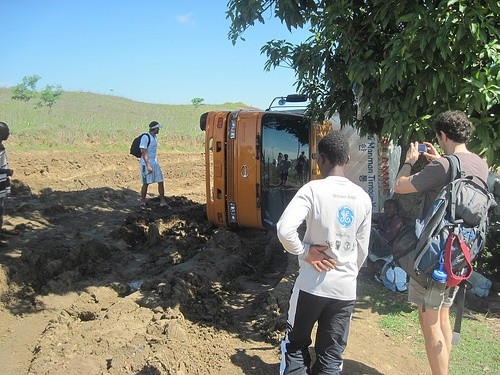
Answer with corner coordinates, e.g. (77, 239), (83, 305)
(371, 198), (404, 256)
(0, 121), (14, 244)
(277, 150), (310, 186)
(394, 110), (489, 375)
(278, 130), (372, 374)
(139, 121), (172, 211)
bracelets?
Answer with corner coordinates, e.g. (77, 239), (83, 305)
(403, 162), (412, 169)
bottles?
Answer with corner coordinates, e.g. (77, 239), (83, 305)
(424, 268), (448, 310)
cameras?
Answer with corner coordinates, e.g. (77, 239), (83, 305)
(418, 144), (427, 152)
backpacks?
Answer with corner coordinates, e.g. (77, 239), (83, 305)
(392, 154), (498, 290)
(130, 133), (151, 159)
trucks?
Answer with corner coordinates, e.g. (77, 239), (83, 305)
(198, 93), (410, 236)
